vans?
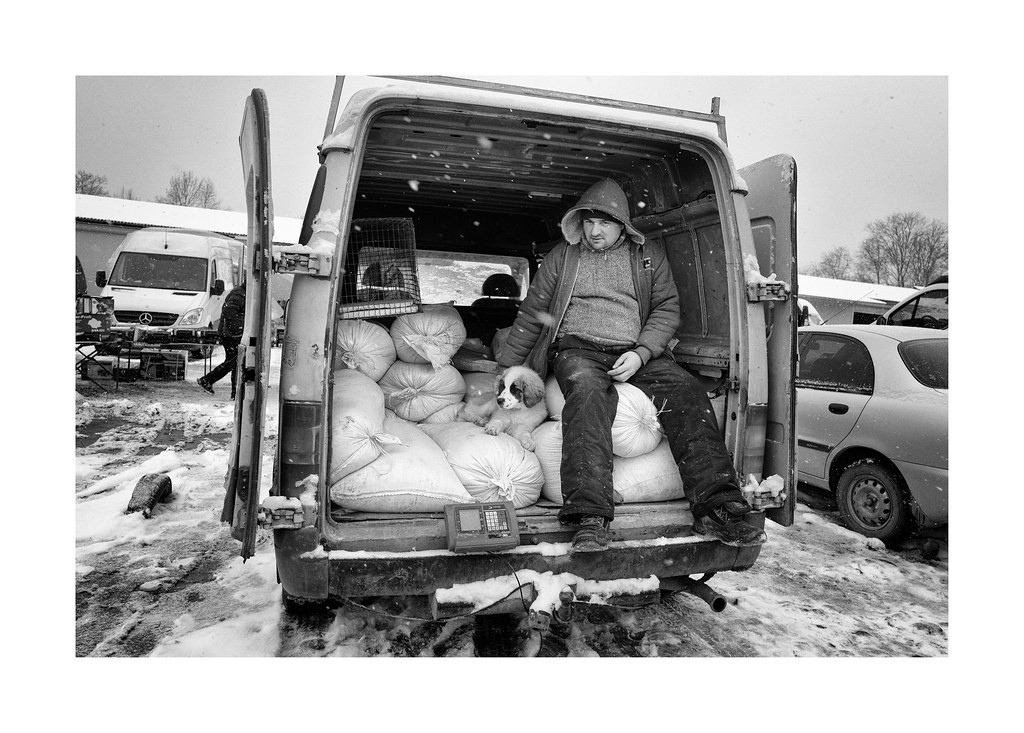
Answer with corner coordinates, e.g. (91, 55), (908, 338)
(94, 228), (248, 359)
(222, 77), (800, 607)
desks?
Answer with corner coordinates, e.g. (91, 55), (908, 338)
(159, 342), (212, 376)
(76, 339), (131, 395)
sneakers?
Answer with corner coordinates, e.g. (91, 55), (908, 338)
(197, 377), (215, 396)
(690, 501), (768, 547)
(230, 393), (235, 400)
(572, 514), (613, 553)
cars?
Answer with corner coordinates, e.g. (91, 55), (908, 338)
(798, 324), (948, 544)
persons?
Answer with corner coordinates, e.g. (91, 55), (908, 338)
(197, 279), (246, 400)
(496, 178), (768, 553)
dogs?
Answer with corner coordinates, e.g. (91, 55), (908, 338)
(456, 364), (550, 452)
